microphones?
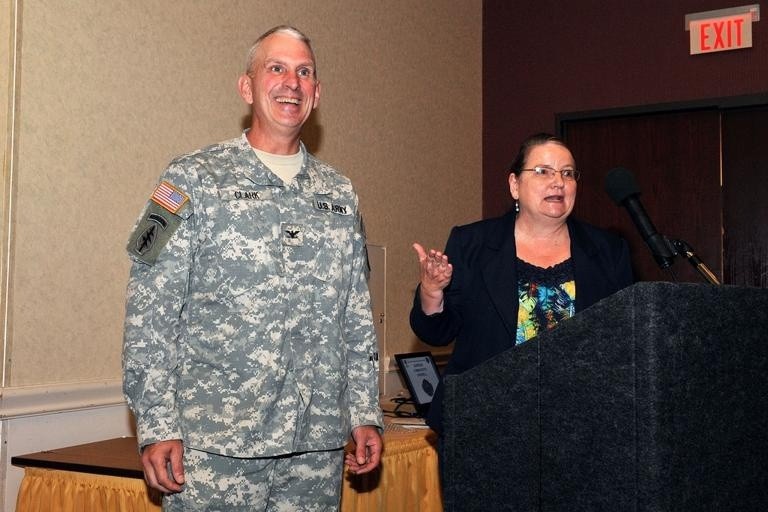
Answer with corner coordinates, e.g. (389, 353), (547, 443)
(603, 167), (679, 284)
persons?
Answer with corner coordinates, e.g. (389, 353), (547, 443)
(409, 133), (636, 436)
(121, 24), (383, 512)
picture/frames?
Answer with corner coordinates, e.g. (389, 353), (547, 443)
(394, 351), (441, 418)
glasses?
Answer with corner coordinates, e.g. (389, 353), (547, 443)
(522, 166), (580, 182)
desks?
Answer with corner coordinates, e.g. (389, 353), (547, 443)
(11, 390), (442, 512)
